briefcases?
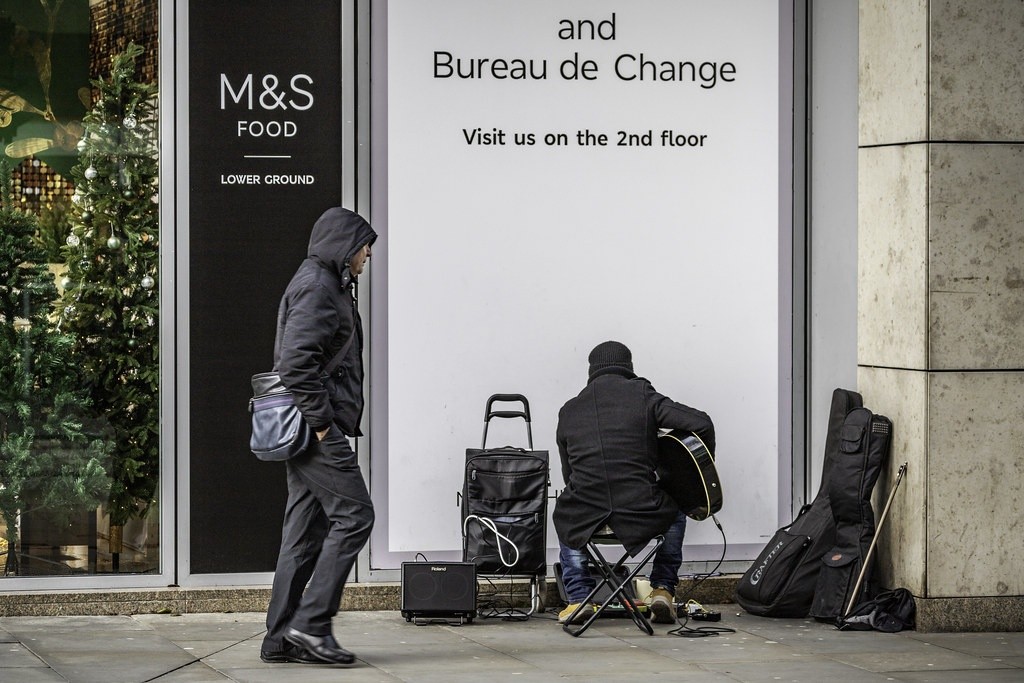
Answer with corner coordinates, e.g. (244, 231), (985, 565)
(554, 560), (652, 619)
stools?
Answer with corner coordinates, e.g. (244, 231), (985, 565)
(561, 529), (665, 637)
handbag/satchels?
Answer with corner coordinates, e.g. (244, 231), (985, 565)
(248, 371), (310, 461)
(839, 588), (916, 633)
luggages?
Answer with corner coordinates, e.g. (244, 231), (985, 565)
(458, 394), (551, 617)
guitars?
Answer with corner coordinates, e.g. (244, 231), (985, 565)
(656, 430), (723, 522)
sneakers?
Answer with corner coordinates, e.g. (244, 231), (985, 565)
(650, 586), (677, 625)
(558, 602), (594, 624)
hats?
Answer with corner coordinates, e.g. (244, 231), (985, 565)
(588, 341), (633, 377)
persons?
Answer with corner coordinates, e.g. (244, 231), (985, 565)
(260, 206), (378, 666)
(556, 340), (717, 623)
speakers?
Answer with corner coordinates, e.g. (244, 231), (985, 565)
(401, 562), (477, 619)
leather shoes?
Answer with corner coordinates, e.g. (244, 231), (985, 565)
(260, 646), (336, 664)
(283, 626), (355, 664)
